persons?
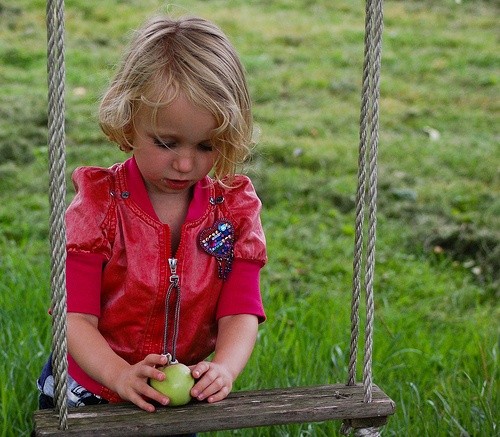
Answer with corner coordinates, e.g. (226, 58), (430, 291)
(34, 18), (267, 409)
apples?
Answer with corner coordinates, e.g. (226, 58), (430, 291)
(149, 360), (194, 406)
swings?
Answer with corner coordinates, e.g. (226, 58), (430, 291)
(28, 0), (395, 437)
